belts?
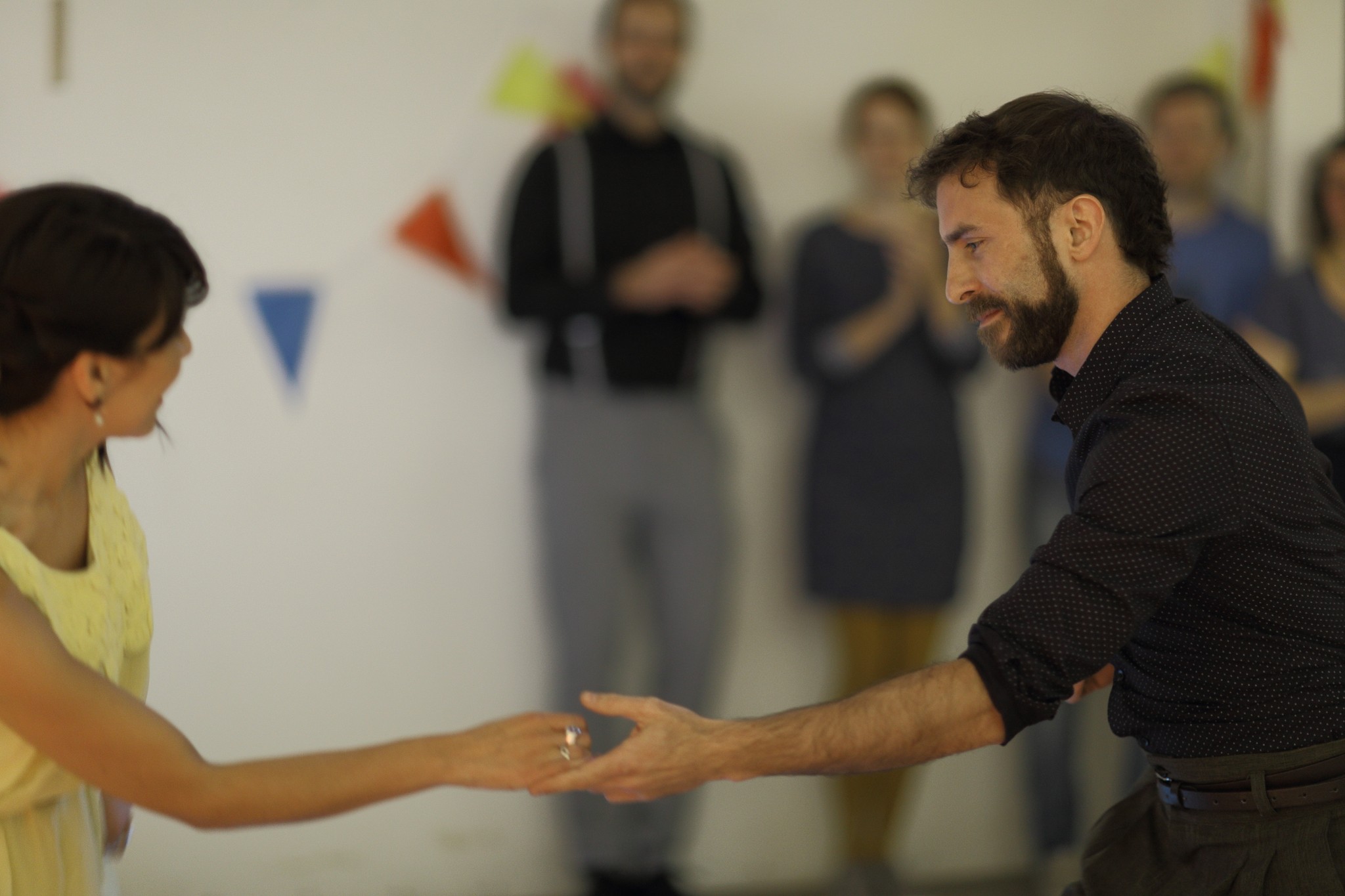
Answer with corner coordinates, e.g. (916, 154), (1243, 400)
(1155, 754), (1345, 812)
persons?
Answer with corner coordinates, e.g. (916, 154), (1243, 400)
(779, 76), (987, 896)
(1014, 71), (1345, 852)
(0, 184), (592, 896)
(533, 93), (1345, 896)
(483, 0), (771, 896)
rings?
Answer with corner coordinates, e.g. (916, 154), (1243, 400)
(567, 725), (582, 744)
(560, 745), (571, 759)
